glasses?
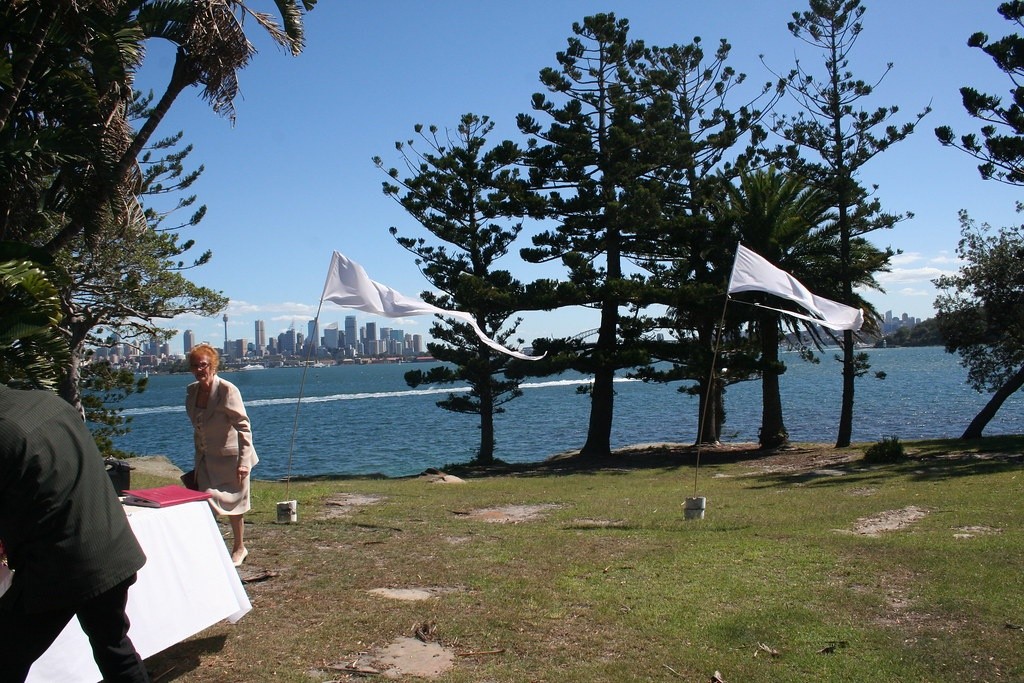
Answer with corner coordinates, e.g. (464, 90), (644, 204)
(189, 363), (212, 371)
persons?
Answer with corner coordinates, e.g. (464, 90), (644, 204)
(186, 345), (259, 566)
(0, 381), (148, 683)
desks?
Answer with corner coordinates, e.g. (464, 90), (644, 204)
(0, 494), (252, 683)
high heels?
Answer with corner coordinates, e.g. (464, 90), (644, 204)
(232, 546), (248, 566)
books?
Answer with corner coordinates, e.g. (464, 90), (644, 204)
(122, 486), (212, 508)
